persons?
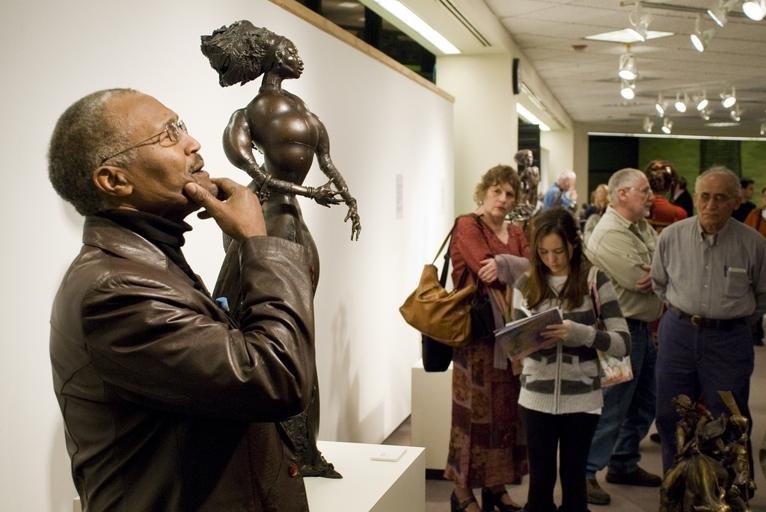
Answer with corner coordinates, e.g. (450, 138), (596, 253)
(440, 158), (765, 511)
(200, 18), (361, 480)
(48, 87), (317, 511)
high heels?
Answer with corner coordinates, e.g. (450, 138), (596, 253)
(481, 485), (522, 512)
(450, 488), (482, 512)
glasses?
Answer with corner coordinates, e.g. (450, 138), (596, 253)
(99, 117), (188, 165)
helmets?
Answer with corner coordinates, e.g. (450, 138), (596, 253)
(646, 159), (679, 193)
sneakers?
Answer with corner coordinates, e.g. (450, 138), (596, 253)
(586, 479), (612, 505)
(605, 464), (664, 488)
(649, 430), (661, 443)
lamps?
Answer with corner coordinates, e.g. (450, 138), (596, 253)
(616, 0), (766, 136)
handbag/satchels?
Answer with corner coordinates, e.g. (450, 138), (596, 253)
(421, 332), (454, 373)
(397, 264), (481, 348)
(591, 316), (634, 388)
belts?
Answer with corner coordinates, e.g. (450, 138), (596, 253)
(667, 302), (754, 330)
